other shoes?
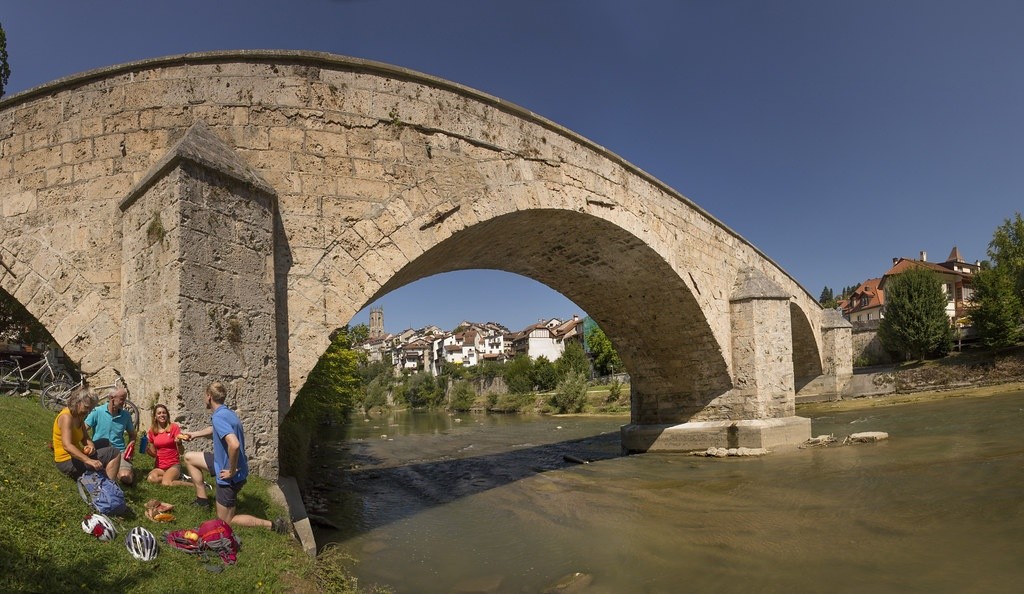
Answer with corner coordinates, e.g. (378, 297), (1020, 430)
(190, 501), (209, 509)
(144, 508), (176, 524)
(179, 473), (191, 480)
(144, 499), (174, 513)
(276, 518), (287, 532)
(203, 482), (212, 490)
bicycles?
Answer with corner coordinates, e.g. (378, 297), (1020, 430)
(41, 368), (139, 433)
(0, 344), (74, 399)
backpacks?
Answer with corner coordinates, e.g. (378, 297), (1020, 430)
(166, 529), (199, 554)
(77, 471), (126, 515)
(196, 520), (240, 565)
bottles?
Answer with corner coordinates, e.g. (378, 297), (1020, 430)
(124, 443), (135, 462)
(139, 434), (148, 454)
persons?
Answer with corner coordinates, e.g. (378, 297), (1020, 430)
(182, 382), (287, 534)
(143, 405), (212, 491)
(53, 391), (124, 495)
(84, 388), (136, 483)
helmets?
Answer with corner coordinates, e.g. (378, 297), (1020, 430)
(126, 526), (158, 561)
(81, 514), (115, 542)
